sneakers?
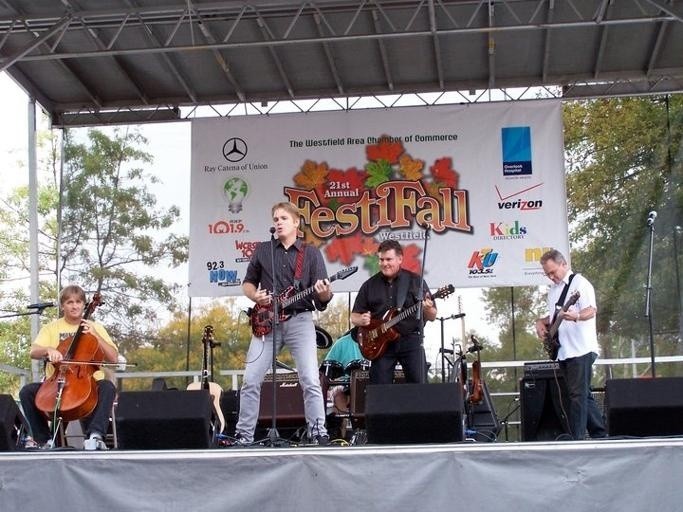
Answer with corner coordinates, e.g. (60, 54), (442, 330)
(311, 434), (331, 446)
(25, 439), (52, 449)
(231, 437), (255, 446)
(90, 436), (107, 450)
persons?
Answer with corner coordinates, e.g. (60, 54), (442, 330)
(534, 248), (607, 442)
(19, 285), (118, 452)
(350, 239), (438, 384)
(324, 330), (371, 446)
(217, 201), (333, 448)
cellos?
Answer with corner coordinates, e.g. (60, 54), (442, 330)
(35, 292), (104, 421)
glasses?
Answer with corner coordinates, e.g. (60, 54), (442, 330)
(544, 263), (560, 277)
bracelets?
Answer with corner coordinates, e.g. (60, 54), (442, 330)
(572, 313), (580, 322)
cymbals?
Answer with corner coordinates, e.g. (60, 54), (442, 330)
(315, 326), (332, 349)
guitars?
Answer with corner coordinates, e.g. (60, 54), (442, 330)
(454, 344), (469, 401)
(542, 291), (579, 360)
(250, 266), (357, 337)
(185, 325), (227, 436)
(470, 336), (484, 405)
(356, 285), (454, 361)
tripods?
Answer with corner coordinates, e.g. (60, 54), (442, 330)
(248, 235), (301, 447)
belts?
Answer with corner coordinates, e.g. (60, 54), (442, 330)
(283, 307), (310, 315)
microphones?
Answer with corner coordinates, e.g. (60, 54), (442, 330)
(422, 222), (434, 229)
(269, 226), (276, 234)
(452, 312), (465, 319)
(646, 210), (657, 228)
(27, 300), (59, 309)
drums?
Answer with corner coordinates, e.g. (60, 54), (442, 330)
(343, 360), (370, 393)
(320, 359), (344, 381)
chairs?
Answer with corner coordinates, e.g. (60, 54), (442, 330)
(60, 367), (123, 450)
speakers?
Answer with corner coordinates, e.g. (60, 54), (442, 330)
(520, 373), (571, 442)
(115, 388), (211, 449)
(0, 394), (30, 452)
(604, 376), (683, 437)
(364, 382), (466, 444)
